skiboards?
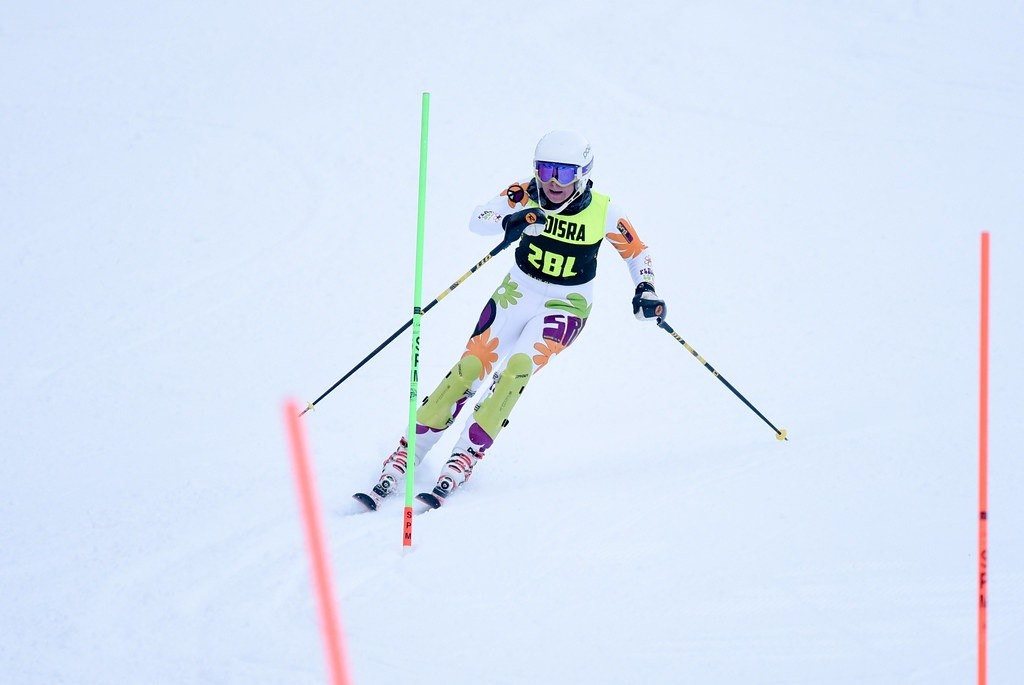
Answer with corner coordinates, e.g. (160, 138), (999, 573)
(413, 491), (442, 509)
(350, 483), (393, 512)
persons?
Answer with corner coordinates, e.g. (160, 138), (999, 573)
(349, 127), (667, 511)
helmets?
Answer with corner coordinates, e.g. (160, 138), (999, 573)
(534, 131), (594, 196)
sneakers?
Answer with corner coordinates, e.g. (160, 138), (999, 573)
(381, 448), (415, 481)
(440, 453), (473, 486)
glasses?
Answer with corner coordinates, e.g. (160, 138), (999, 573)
(535, 161), (581, 188)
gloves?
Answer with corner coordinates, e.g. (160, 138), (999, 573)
(509, 208), (548, 237)
(632, 288), (666, 325)
